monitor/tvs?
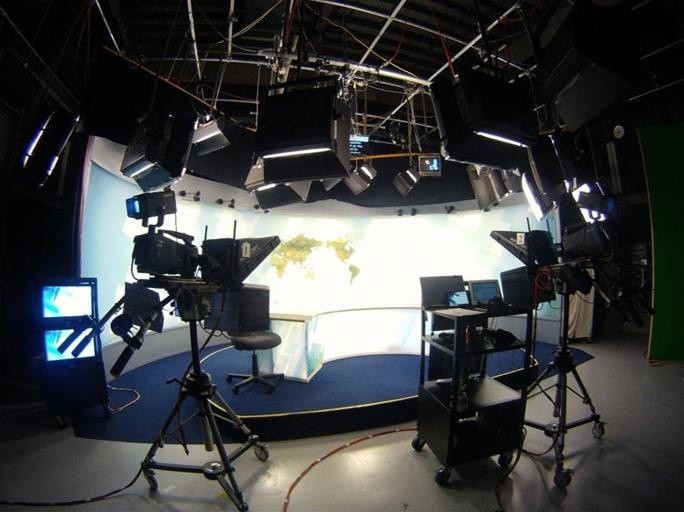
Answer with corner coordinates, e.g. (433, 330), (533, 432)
(40, 283), (94, 320)
(418, 156), (441, 177)
(347, 134), (371, 157)
(444, 289), (471, 308)
(469, 280), (503, 307)
(42, 326), (99, 363)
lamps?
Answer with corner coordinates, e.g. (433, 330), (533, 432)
(417, 154), (443, 178)
(429, 71), (531, 171)
(121, 109), (200, 193)
(192, 114), (241, 157)
(463, 163), (523, 215)
(521, 172), (550, 223)
(125, 188), (178, 221)
(344, 165), (377, 195)
(255, 85), (354, 186)
(394, 165), (420, 197)
(244, 155), (314, 215)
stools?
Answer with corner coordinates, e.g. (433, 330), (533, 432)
(225, 333), (285, 394)
